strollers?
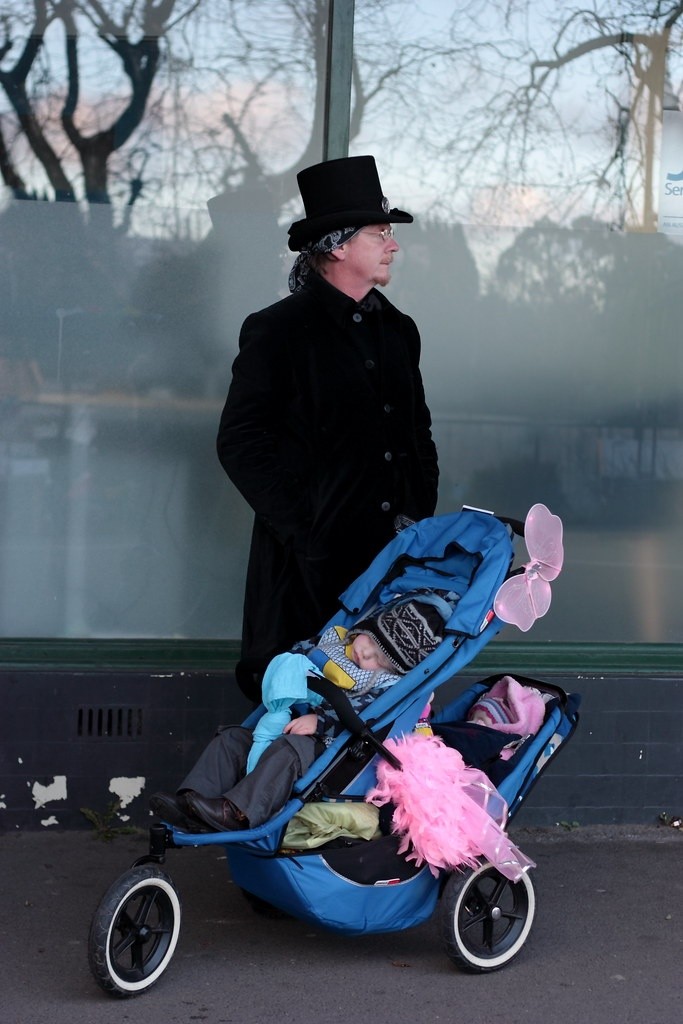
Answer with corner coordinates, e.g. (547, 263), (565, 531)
(87, 507), (584, 1001)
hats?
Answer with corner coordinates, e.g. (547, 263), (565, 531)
(288, 155), (414, 251)
(317, 599), (446, 698)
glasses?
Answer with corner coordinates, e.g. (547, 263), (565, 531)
(359, 227), (394, 241)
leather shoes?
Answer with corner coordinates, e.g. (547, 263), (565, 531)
(150, 793), (215, 834)
(185, 790), (249, 832)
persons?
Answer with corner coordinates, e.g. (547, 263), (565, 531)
(320, 676), (546, 885)
(147, 583), (459, 832)
(213, 153), (440, 737)
